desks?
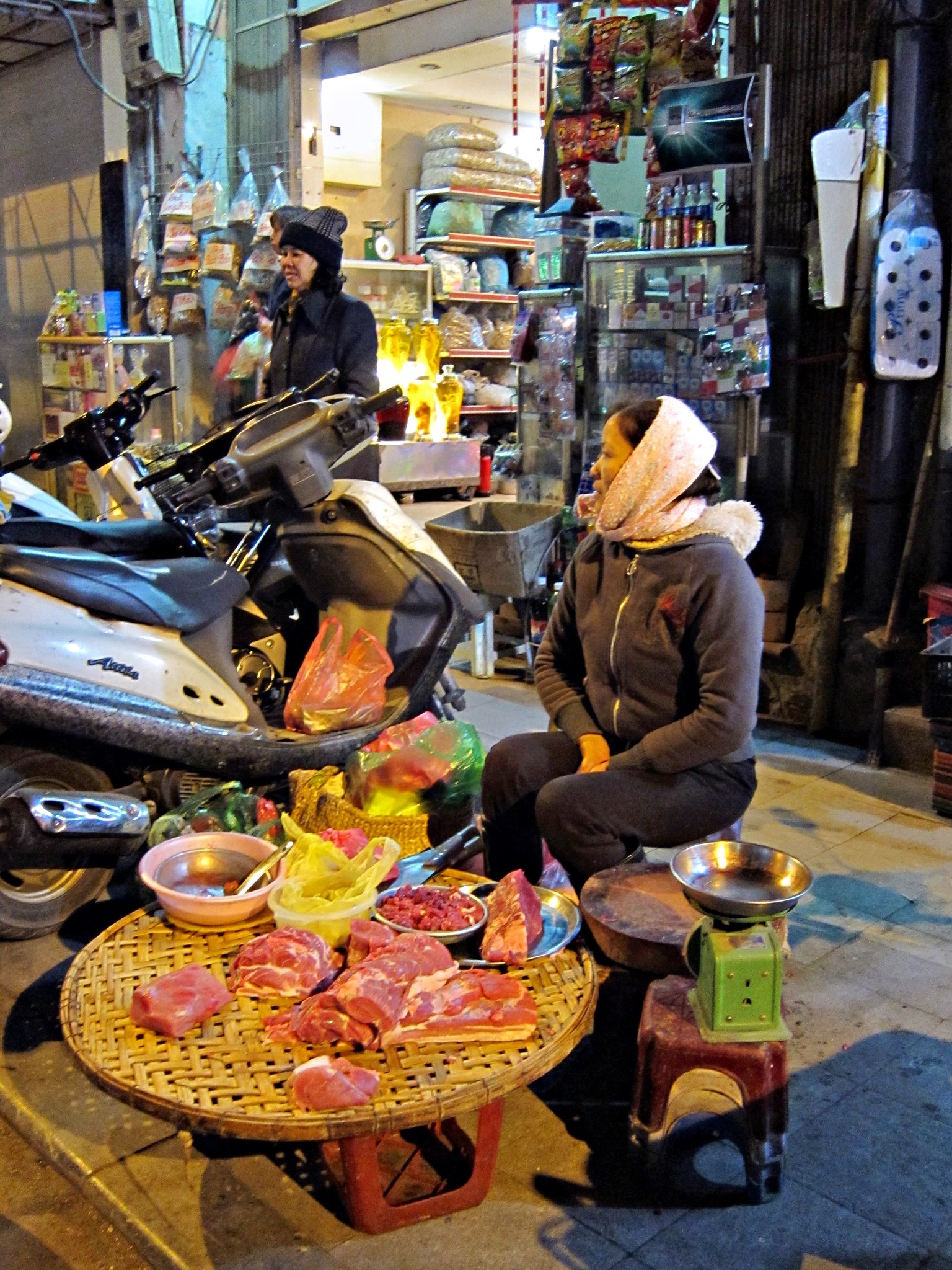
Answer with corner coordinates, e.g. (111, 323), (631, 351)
(54, 859), (602, 1225)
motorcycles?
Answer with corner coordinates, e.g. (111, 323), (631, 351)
(0, 365), (480, 938)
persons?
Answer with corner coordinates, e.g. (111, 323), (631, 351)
(260, 208), (309, 343)
(477, 393), (765, 908)
(260, 206), (380, 409)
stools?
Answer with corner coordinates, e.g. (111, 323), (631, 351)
(625, 980), (794, 1203)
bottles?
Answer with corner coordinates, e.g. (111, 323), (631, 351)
(437, 364), (463, 440)
(530, 460), (595, 646)
(406, 375), (436, 443)
(378, 314), (412, 373)
(656, 184), (712, 217)
(412, 317), (443, 383)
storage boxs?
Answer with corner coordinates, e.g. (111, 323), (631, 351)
(430, 496), (570, 596)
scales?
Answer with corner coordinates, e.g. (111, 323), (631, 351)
(363, 219), (396, 261)
(669, 838), (815, 1046)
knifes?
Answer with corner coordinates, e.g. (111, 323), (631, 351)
(377, 822), (483, 891)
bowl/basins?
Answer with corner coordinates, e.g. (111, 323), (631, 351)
(267, 876), (377, 950)
(135, 829), (287, 928)
(371, 882), (487, 947)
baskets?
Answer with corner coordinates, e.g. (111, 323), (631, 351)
(288, 763), (431, 863)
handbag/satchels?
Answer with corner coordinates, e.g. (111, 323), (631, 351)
(283, 616), (395, 735)
(212, 330), (274, 400)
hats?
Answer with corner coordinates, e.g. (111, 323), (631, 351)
(278, 204), (348, 271)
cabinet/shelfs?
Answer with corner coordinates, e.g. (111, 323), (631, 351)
(37, 329), (183, 525)
(581, 241), (766, 518)
(402, 186), (543, 416)
(336, 256), (435, 369)
